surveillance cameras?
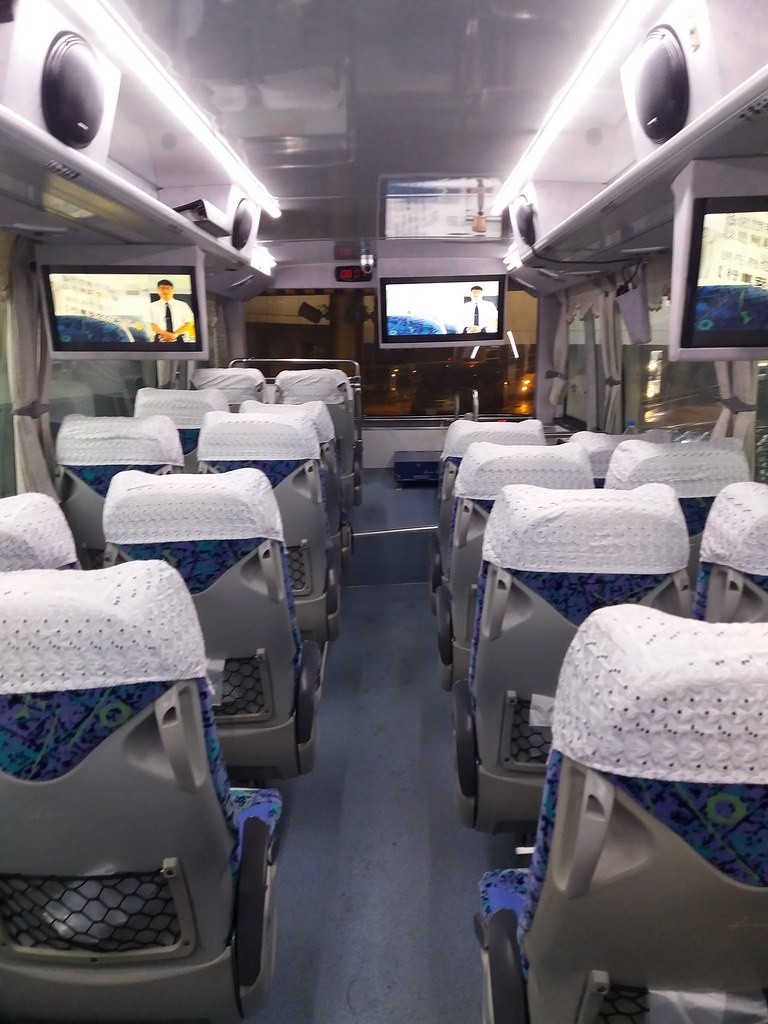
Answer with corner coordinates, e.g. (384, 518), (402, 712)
(360, 259), (375, 273)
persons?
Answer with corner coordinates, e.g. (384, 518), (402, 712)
(147, 279), (194, 343)
(461, 286), (498, 334)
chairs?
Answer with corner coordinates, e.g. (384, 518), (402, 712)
(0, 367), (768, 1024)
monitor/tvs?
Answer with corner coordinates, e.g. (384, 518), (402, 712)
(669, 160), (768, 361)
(35, 245), (209, 361)
(378, 274), (507, 348)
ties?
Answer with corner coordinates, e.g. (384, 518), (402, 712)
(165, 303), (173, 333)
(474, 304), (479, 326)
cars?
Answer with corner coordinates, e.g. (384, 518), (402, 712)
(410, 363), (505, 415)
